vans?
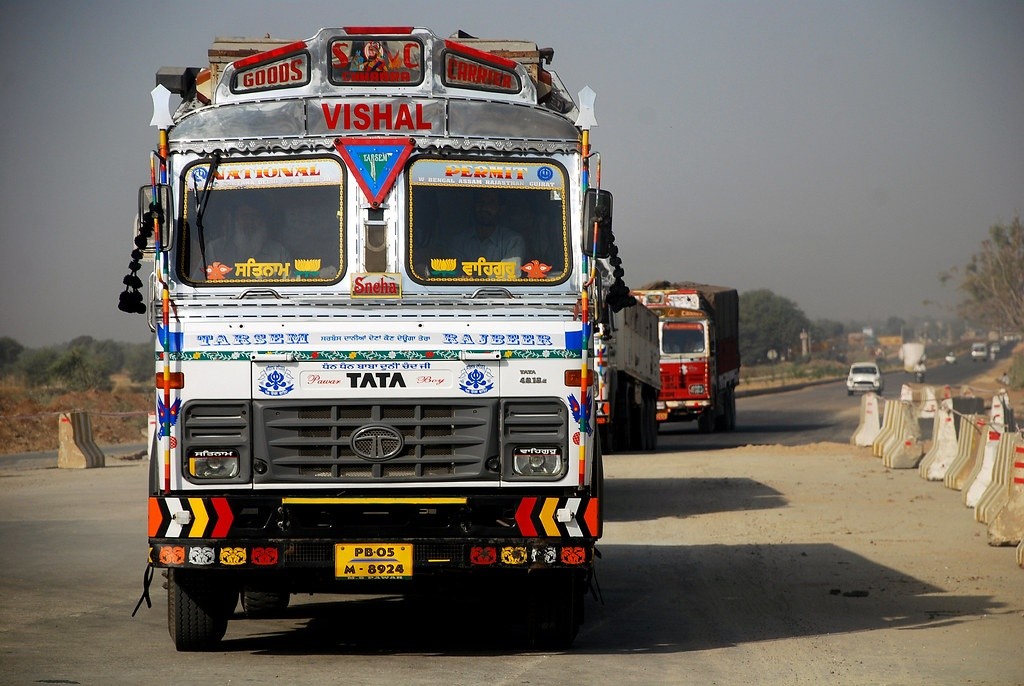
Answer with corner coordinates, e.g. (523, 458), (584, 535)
(970, 342), (987, 362)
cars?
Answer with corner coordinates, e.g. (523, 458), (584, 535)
(845, 360), (882, 397)
(945, 353), (956, 365)
(990, 344), (999, 354)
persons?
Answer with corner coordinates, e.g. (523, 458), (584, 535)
(445, 190), (525, 278)
(191, 200), (294, 281)
(664, 334), (698, 352)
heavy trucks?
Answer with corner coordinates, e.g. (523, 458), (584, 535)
(119, 22), (633, 647)
(627, 279), (740, 435)
(592, 276), (661, 453)
